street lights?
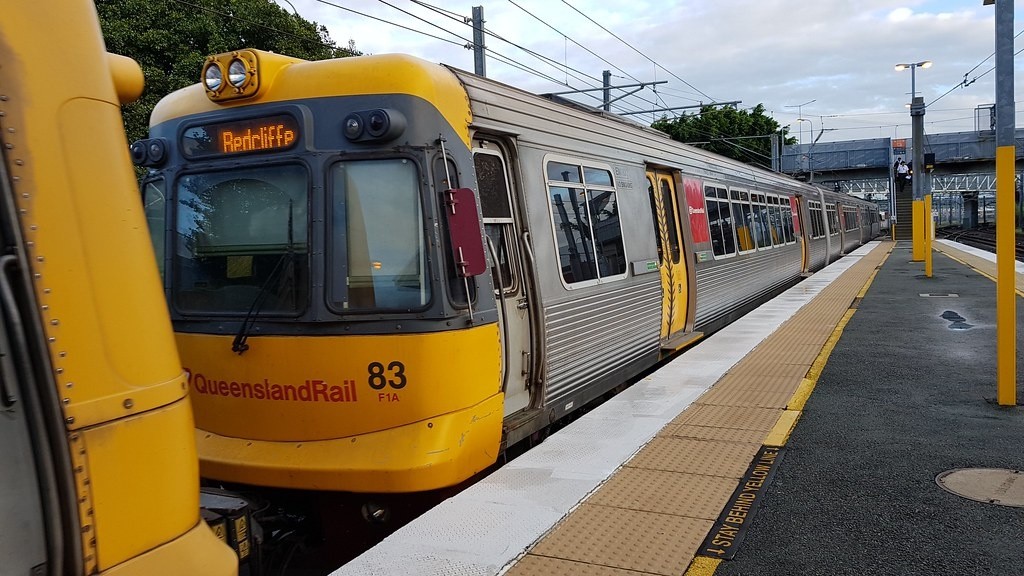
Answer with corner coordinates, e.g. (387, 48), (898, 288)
(797, 118), (812, 169)
(783, 99), (818, 143)
(894, 60), (933, 99)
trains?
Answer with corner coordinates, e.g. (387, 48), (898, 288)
(129, 50), (881, 525)
(2, 0), (243, 576)
